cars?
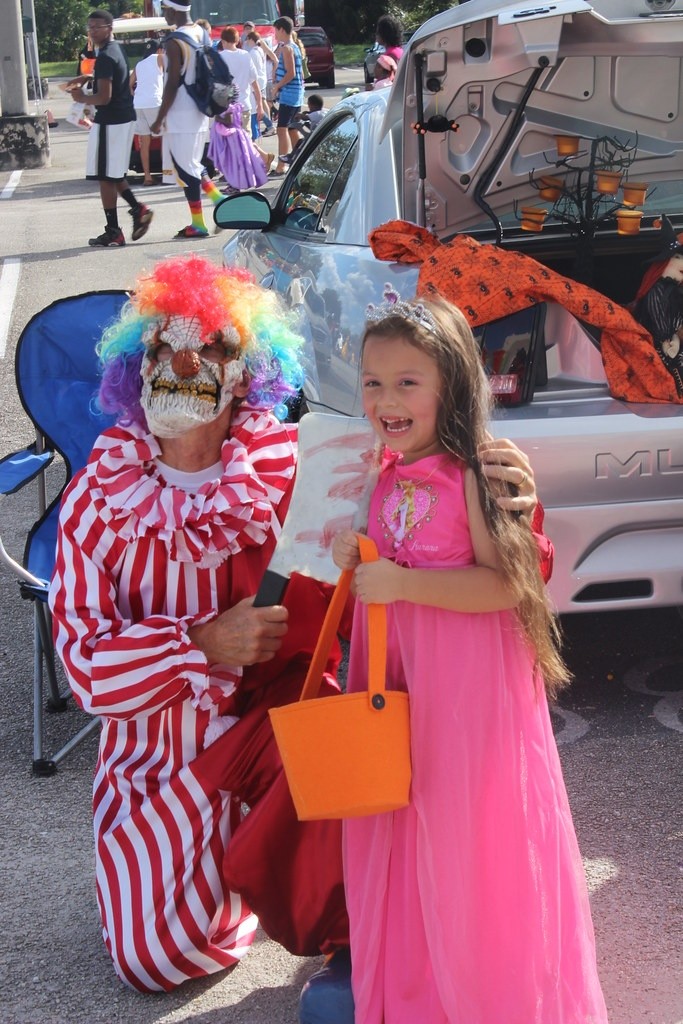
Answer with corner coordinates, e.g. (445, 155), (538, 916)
(112, 16), (176, 173)
(214, 0), (683, 616)
(364, 32), (414, 83)
(293, 27), (335, 88)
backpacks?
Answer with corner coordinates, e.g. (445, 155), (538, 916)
(165, 25), (235, 117)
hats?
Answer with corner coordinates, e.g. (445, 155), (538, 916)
(376, 55), (397, 71)
(142, 39), (160, 59)
(341, 88), (360, 100)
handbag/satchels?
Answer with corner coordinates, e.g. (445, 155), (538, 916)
(66, 79), (96, 129)
(268, 533), (412, 820)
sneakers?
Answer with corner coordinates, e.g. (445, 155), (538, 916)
(88, 226), (126, 246)
(128, 201), (153, 241)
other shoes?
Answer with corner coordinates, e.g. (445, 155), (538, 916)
(219, 175), (227, 183)
(274, 112), (279, 121)
(265, 153), (274, 173)
(214, 226), (222, 235)
(279, 154), (293, 164)
(268, 170), (284, 177)
(210, 170), (217, 179)
(143, 178), (158, 186)
(219, 187), (240, 196)
(263, 127), (276, 137)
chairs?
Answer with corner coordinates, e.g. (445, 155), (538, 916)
(0, 289), (136, 779)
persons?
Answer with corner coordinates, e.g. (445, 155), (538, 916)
(300, 289), (605, 1024)
(62, 0), (331, 247)
(42, 259), (543, 991)
(366, 15), (407, 91)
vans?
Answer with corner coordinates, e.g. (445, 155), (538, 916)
(144, 0), (305, 50)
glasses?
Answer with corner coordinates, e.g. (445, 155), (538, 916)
(85, 24), (111, 31)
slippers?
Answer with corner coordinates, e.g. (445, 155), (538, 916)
(174, 225), (209, 238)
